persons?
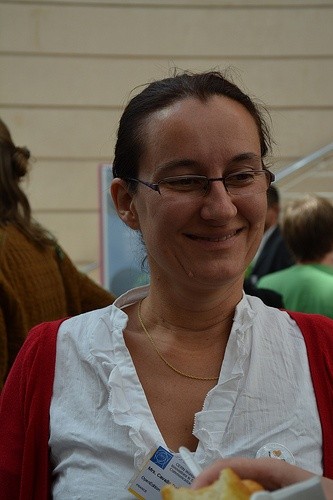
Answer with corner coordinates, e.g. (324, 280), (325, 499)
(1, 72), (332, 500)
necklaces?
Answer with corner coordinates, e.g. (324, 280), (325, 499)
(137, 296), (220, 381)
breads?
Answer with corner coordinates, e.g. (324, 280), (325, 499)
(160, 468), (265, 500)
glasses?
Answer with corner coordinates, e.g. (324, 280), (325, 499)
(125, 166), (277, 200)
